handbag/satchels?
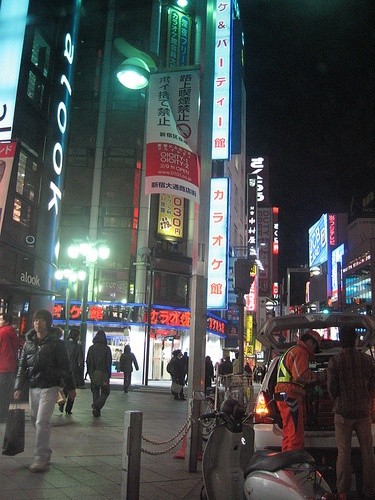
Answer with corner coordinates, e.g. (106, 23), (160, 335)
(170, 380), (182, 393)
(2, 399), (26, 456)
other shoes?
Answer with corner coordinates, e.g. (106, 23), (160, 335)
(91, 403), (101, 416)
(174, 396), (180, 400)
(180, 397), (187, 401)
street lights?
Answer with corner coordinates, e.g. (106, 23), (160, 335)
(285, 266), (321, 342)
(54, 265), (87, 343)
(66, 233), (110, 378)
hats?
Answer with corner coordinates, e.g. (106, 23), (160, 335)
(307, 329), (323, 353)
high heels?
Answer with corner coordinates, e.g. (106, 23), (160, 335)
(65, 409), (73, 414)
(57, 404), (64, 412)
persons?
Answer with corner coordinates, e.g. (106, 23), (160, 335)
(86, 330), (112, 417)
(13, 310), (77, 473)
(0, 313), (19, 423)
(273, 330), (323, 452)
(119, 345), (139, 393)
(326, 325), (375, 500)
(166, 349), (266, 401)
(58, 328), (85, 415)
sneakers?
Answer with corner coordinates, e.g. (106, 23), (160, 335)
(29, 457), (51, 472)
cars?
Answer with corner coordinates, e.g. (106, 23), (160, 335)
(253, 311), (375, 457)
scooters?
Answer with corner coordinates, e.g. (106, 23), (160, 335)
(197, 370), (263, 442)
(196, 391), (338, 500)
